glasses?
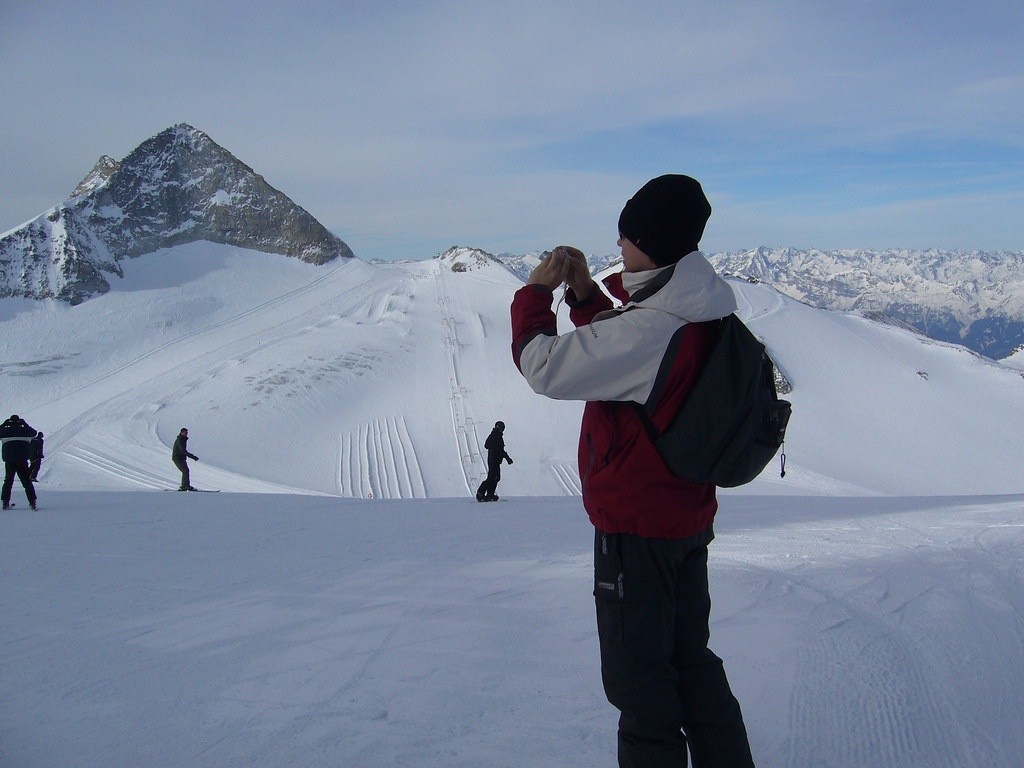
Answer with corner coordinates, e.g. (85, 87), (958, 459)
(618, 230), (624, 240)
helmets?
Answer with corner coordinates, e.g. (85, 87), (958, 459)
(494, 421), (505, 431)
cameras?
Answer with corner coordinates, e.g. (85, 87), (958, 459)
(538, 250), (569, 261)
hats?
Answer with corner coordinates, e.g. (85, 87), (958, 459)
(616, 173), (713, 260)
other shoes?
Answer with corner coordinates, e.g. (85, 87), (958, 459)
(30, 503), (38, 510)
(487, 493), (499, 501)
(476, 490), (490, 502)
(188, 486), (197, 491)
(31, 478), (39, 482)
(2, 504), (10, 509)
(178, 486), (188, 491)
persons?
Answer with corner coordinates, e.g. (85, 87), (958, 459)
(172, 428), (199, 491)
(28, 432), (45, 482)
(476, 421), (513, 502)
(510, 174), (755, 768)
(0, 415), (37, 510)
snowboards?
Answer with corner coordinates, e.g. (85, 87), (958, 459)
(466, 496), (510, 506)
(163, 486), (223, 495)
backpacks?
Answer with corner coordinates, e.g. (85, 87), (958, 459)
(634, 310), (793, 487)
